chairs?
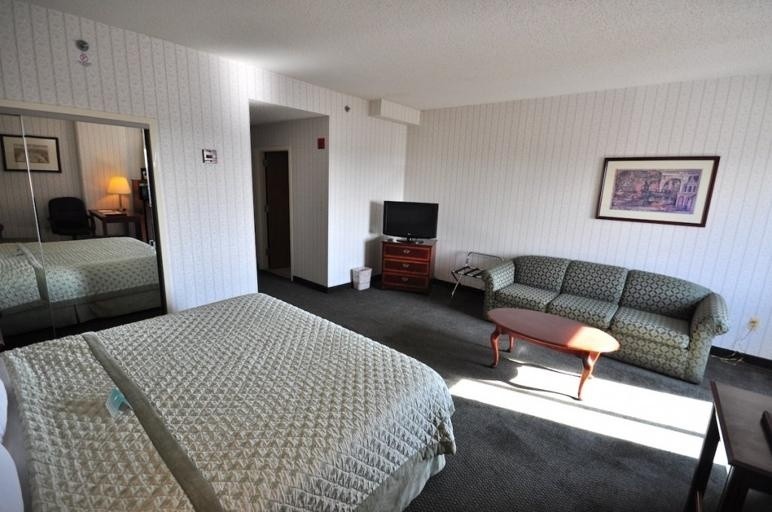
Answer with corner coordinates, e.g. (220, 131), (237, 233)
(45, 197), (95, 239)
(446, 251), (504, 306)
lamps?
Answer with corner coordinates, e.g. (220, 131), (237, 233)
(106, 176), (132, 213)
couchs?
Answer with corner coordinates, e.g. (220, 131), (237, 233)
(479, 254), (730, 385)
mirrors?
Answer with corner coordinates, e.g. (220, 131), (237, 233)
(0, 97), (174, 353)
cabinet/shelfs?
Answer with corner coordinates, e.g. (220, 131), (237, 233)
(379, 237), (437, 296)
(131, 179), (154, 245)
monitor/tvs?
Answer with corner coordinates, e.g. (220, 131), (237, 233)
(382, 200), (439, 245)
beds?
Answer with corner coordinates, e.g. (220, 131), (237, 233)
(0, 291), (459, 512)
(0, 236), (163, 337)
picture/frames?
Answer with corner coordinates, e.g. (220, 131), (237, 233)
(0, 134), (61, 173)
(140, 167), (147, 181)
(595, 155), (720, 228)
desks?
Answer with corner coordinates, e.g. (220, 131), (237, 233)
(88, 208), (144, 242)
(683, 380), (771, 512)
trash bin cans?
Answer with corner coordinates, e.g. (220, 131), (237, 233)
(352, 267), (372, 291)
